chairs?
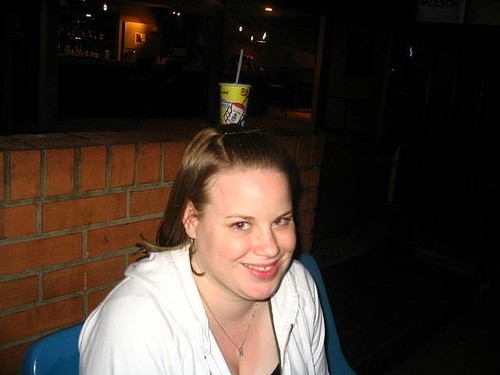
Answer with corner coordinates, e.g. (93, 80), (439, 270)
(23, 254), (355, 375)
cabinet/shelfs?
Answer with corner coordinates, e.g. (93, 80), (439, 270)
(158, 8), (221, 67)
(56, 6), (117, 62)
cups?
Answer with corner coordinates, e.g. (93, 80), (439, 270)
(219, 82), (251, 126)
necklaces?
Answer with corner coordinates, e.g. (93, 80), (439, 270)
(193, 273), (258, 357)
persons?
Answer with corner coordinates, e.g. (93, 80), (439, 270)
(77, 127), (331, 375)
